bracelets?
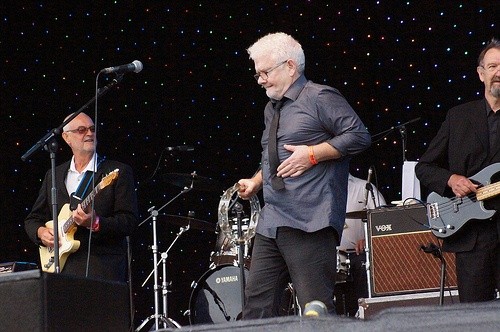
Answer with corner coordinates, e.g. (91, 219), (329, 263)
(87, 216), (99, 229)
(308, 146), (318, 164)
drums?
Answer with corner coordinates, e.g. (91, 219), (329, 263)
(186, 263), (250, 327)
(209, 217), (255, 267)
(336, 249), (351, 284)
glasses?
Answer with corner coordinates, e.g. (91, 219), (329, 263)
(254, 60), (287, 81)
(67, 125), (96, 135)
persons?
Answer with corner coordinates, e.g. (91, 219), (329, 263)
(337, 173), (387, 255)
(237, 32), (373, 321)
(24, 113), (139, 282)
(415, 42), (500, 303)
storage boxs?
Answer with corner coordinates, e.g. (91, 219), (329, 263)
(0, 270), (130, 332)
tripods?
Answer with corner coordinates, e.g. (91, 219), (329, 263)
(136, 183), (195, 330)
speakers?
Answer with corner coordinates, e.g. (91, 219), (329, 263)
(1, 271), (134, 332)
(366, 204), (459, 296)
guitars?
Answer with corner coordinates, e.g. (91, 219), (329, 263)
(39, 169), (119, 273)
(426, 163), (500, 237)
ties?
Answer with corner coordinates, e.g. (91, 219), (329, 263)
(268, 96), (289, 191)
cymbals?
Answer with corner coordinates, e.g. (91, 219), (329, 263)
(158, 172), (219, 192)
(157, 212), (213, 230)
(217, 183), (261, 242)
(345, 211), (367, 219)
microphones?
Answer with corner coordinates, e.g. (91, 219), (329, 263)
(168, 145), (196, 151)
(102, 60), (144, 74)
(363, 169), (373, 205)
